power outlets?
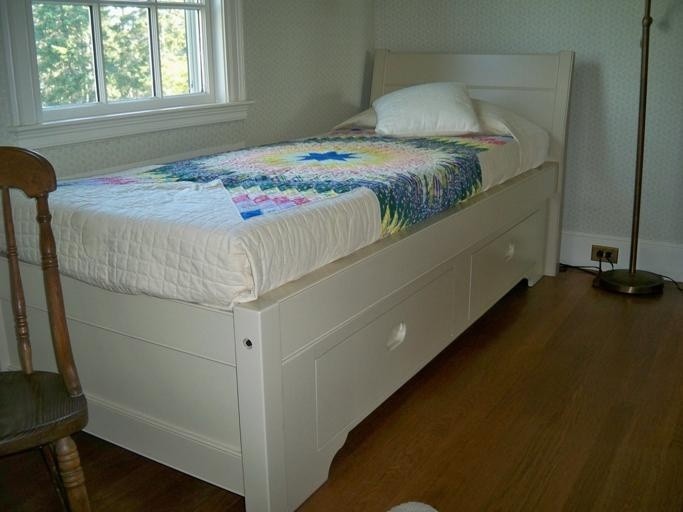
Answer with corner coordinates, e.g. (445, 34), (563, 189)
(592, 246), (619, 264)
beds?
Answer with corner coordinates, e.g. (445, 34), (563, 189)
(0, 50), (576, 512)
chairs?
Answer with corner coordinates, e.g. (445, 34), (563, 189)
(0, 146), (91, 512)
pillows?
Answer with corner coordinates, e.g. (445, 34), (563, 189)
(372, 82), (481, 136)
